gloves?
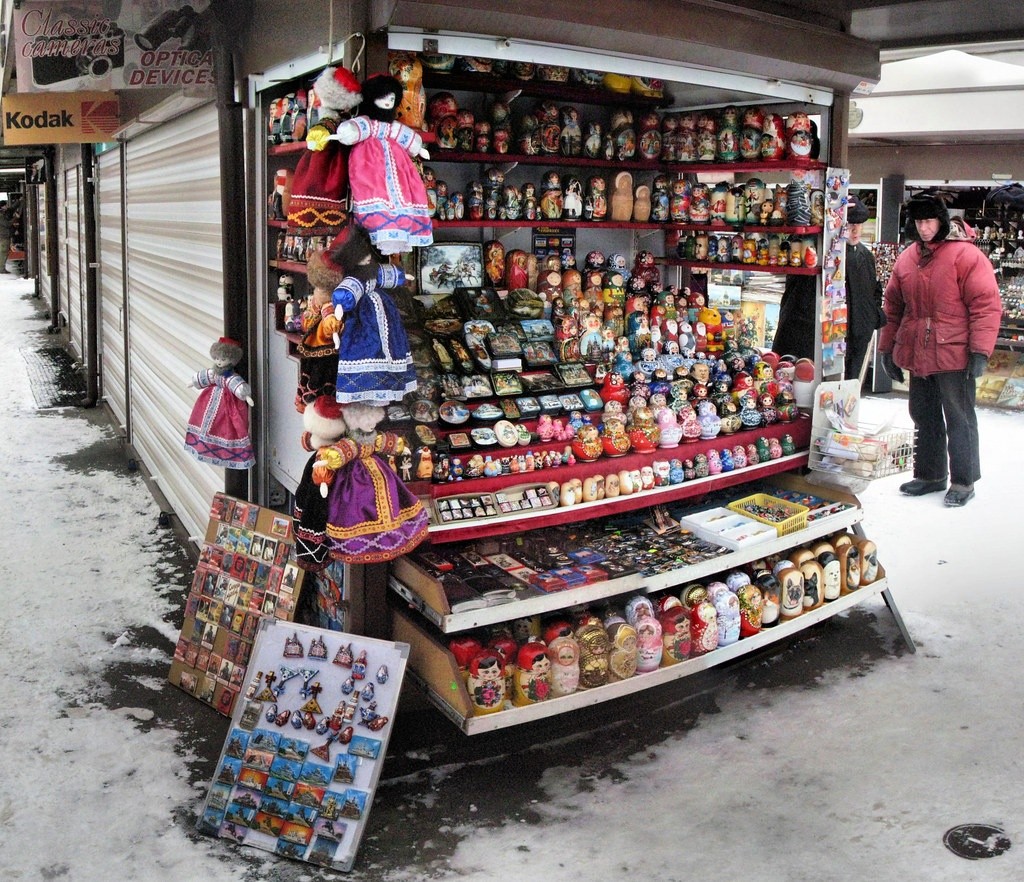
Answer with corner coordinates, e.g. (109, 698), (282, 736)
(881, 353), (905, 383)
(966, 353), (988, 380)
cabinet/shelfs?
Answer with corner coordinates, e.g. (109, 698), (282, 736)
(246, 25), (891, 739)
(970, 225), (1024, 350)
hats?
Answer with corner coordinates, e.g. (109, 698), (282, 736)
(902, 191), (949, 244)
(847, 195), (871, 224)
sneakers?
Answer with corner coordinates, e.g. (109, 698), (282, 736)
(944, 483), (974, 507)
(899, 478), (948, 496)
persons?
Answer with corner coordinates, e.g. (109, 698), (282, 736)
(183, 54), (434, 565)
(876, 191), (1002, 507)
(769, 196), (888, 381)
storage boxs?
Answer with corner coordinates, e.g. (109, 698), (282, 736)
(679, 507), (777, 551)
